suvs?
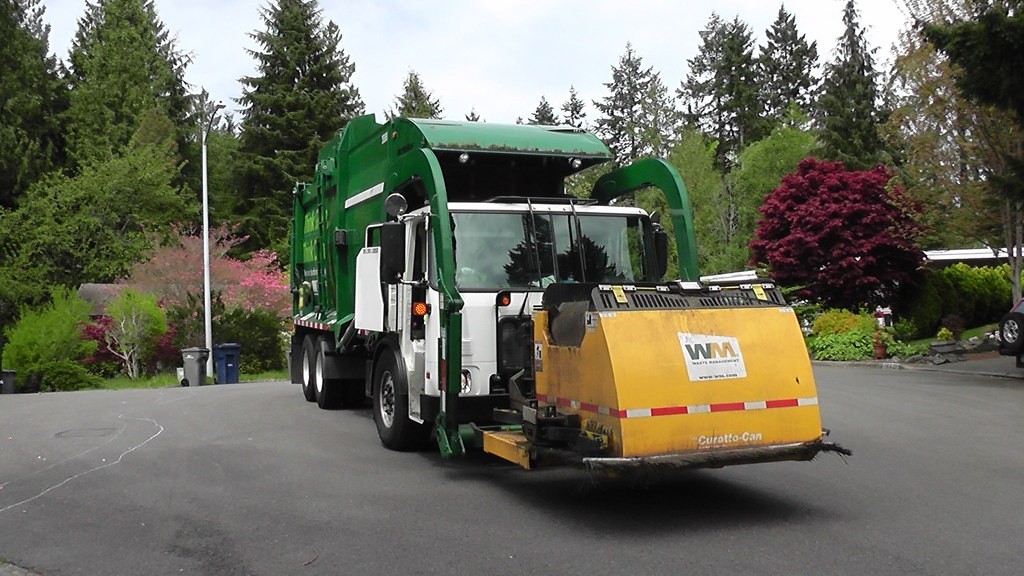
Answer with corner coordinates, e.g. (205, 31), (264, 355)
(998, 295), (1024, 370)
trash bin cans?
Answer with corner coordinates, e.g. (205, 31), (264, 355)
(180, 346), (211, 387)
(213, 343), (241, 385)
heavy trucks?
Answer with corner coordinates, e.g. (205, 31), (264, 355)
(286, 113), (821, 477)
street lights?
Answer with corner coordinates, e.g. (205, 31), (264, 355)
(202, 100), (228, 384)
